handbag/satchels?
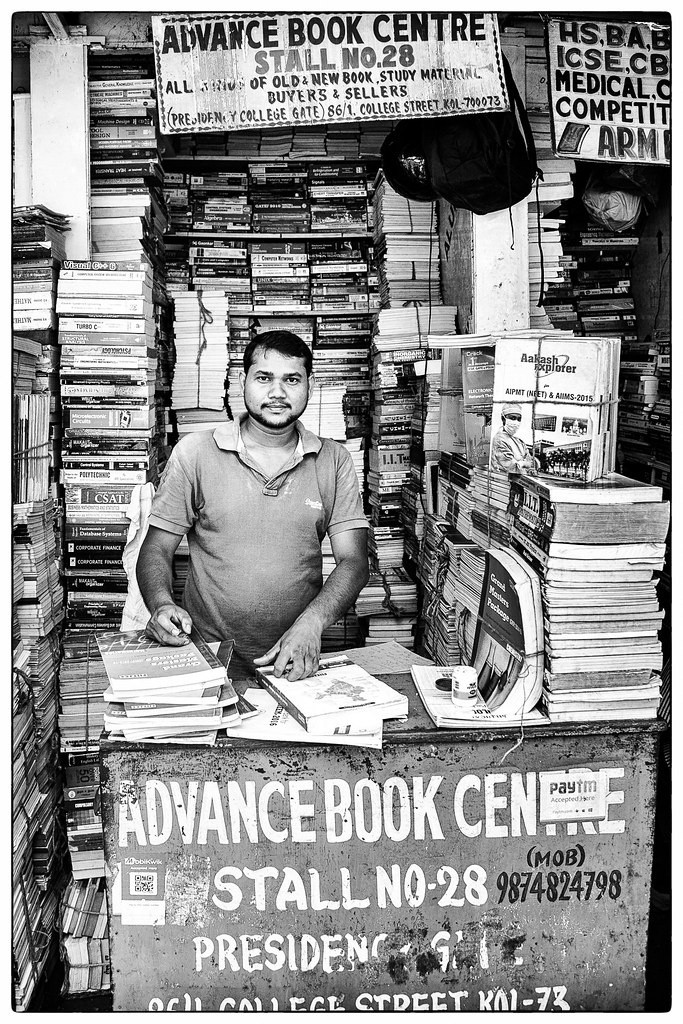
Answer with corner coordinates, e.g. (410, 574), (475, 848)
(379, 46), (536, 216)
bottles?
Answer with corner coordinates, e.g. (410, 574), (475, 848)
(451, 666), (478, 707)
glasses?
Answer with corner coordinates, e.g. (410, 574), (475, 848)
(506, 416), (521, 421)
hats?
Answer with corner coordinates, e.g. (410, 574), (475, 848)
(501, 402), (522, 416)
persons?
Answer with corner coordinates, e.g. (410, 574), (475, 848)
(136, 330), (370, 682)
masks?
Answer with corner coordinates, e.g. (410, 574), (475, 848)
(501, 412), (520, 436)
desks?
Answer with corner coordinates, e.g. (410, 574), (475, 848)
(99, 674), (668, 1011)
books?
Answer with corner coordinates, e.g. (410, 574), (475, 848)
(11, 11), (672, 1014)
